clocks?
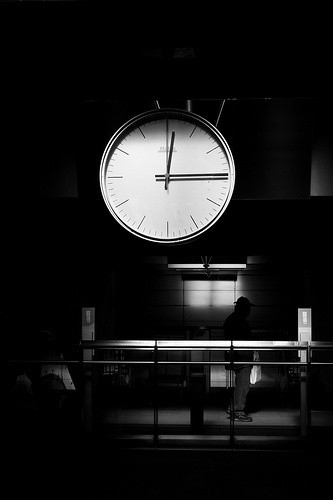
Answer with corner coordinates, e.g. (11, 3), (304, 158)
(99, 109), (236, 243)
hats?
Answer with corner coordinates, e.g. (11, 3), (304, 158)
(236, 296), (256, 307)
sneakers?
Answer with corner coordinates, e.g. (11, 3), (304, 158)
(226, 409), (252, 422)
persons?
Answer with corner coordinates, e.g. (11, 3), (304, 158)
(220, 297), (260, 422)
